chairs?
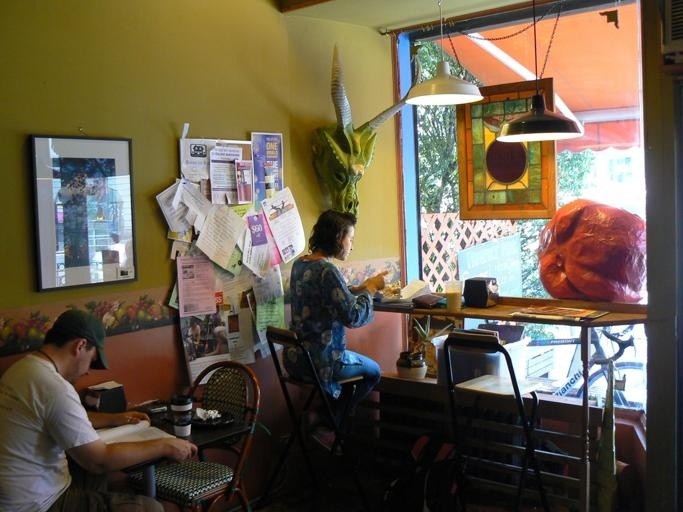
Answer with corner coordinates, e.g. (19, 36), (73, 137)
(438, 328), (552, 512)
(128, 361), (260, 512)
(259, 327), (366, 509)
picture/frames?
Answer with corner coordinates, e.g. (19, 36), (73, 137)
(27, 136), (137, 291)
(456, 77), (556, 220)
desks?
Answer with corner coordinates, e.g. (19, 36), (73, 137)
(372, 291), (648, 512)
(65, 399), (251, 498)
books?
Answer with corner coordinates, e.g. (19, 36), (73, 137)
(94, 419), (177, 444)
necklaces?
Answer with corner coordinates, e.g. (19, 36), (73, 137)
(33, 348), (60, 374)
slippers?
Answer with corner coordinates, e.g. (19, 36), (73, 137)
(309, 430), (345, 458)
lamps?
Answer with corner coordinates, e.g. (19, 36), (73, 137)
(405, 1), (483, 105)
(497, 1), (585, 143)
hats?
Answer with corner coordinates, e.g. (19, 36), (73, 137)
(53, 308), (110, 370)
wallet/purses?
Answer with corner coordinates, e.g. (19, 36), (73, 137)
(412, 293), (449, 309)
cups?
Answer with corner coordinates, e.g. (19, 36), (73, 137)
(169, 393), (193, 436)
(445, 280), (462, 311)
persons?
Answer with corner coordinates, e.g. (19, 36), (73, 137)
(283, 209), (389, 456)
(0, 310), (198, 512)
(187, 317), (201, 359)
